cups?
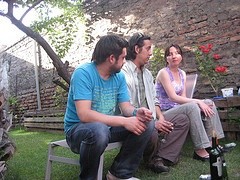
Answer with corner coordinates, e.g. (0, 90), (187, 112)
(221, 88), (233, 98)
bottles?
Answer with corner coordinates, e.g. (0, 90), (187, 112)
(209, 137), (224, 180)
(215, 137), (228, 180)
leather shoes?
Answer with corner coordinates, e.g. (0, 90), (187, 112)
(193, 150), (210, 162)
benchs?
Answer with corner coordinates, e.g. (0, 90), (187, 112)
(44, 139), (161, 180)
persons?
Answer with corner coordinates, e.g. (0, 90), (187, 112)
(155, 44), (229, 162)
(63, 35), (155, 180)
(113, 34), (191, 173)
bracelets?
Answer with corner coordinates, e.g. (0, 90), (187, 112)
(132, 108), (138, 116)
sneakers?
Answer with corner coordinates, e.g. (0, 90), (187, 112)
(106, 170), (141, 180)
(148, 158), (169, 174)
